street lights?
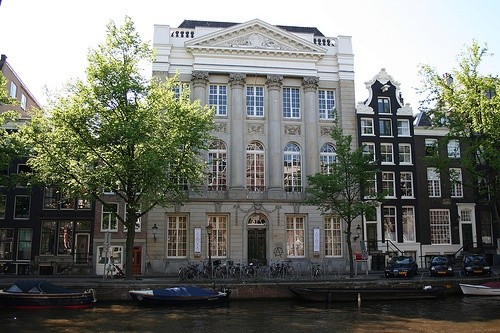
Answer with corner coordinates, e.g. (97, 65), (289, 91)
(205, 223), (213, 266)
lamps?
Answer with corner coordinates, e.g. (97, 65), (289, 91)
(152, 224), (158, 241)
(353, 224), (361, 241)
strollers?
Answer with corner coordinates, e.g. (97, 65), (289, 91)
(113, 264), (125, 279)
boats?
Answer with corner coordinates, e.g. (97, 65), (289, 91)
(128, 285), (231, 308)
(0, 278), (98, 311)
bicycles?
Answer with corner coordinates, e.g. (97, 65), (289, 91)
(268, 260), (295, 281)
(306, 262), (323, 278)
(178, 259), (260, 283)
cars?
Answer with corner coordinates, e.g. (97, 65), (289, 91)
(427, 255), (454, 277)
(384, 256), (418, 278)
(461, 253), (491, 277)
